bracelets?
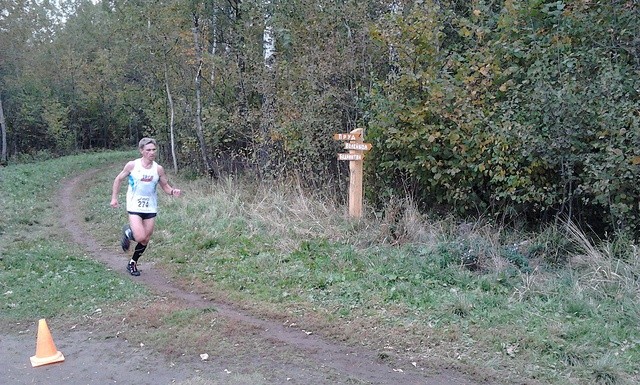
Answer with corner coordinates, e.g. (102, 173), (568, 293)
(171, 190), (173, 195)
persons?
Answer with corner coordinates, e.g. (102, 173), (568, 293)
(111, 137), (181, 276)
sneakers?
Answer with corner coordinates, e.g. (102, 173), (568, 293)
(126, 261), (140, 276)
(122, 228), (131, 252)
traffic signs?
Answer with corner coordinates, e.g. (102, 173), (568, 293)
(333, 133), (372, 161)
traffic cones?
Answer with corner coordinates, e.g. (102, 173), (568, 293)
(29, 318), (65, 367)
